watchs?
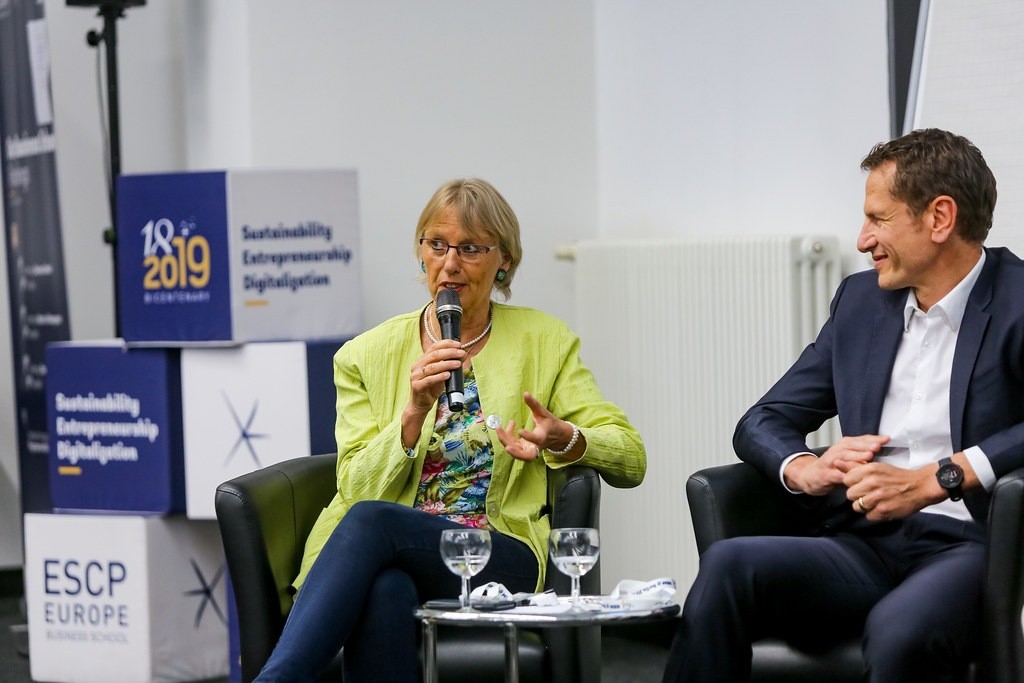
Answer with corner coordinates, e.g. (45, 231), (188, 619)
(936, 458), (965, 501)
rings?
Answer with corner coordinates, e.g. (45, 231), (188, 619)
(536, 448), (540, 458)
(422, 366), (425, 375)
(858, 498), (869, 512)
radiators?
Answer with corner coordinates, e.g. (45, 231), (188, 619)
(556, 229), (844, 602)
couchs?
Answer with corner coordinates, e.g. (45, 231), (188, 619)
(215, 453), (602, 683)
(686, 445), (1024, 683)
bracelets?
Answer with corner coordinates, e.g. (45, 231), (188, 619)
(546, 421), (580, 454)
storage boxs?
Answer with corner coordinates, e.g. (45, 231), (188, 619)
(115, 167), (361, 351)
(23, 508), (231, 683)
(184, 334), (354, 522)
(45, 338), (183, 514)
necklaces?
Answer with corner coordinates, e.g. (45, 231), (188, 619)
(424, 302), (493, 355)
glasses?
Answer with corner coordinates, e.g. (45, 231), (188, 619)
(419, 230), (500, 262)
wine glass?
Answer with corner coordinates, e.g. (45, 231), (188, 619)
(440, 528), (491, 613)
(550, 528), (600, 618)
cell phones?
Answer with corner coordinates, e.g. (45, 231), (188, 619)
(426, 599), (517, 611)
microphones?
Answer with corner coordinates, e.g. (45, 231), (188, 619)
(435, 288), (465, 412)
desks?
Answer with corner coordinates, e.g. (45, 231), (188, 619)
(411, 593), (680, 683)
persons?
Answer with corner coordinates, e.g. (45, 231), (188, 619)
(249, 175), (646, 683)
(665, 129), (1024, 683)
